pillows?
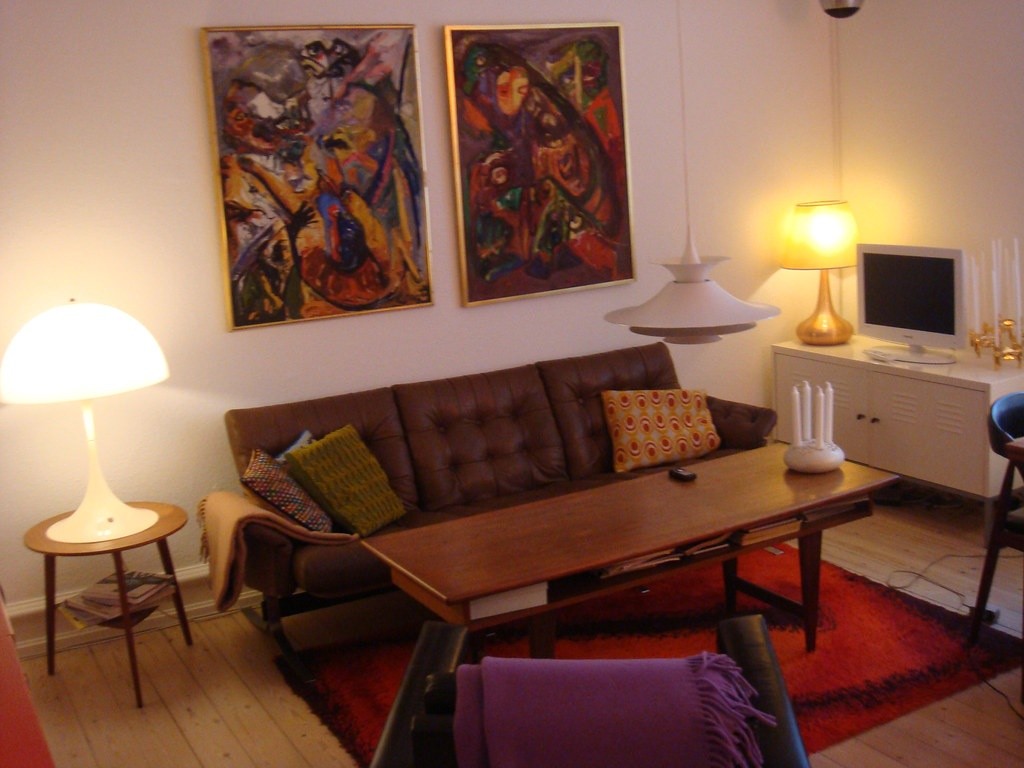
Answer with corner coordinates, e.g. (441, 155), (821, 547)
(283, 421), (407, 538)
(239, 447), (333, 532)
(272, 428), (318, 472)
(600, 388), (720, 476)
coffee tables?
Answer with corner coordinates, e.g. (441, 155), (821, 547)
(370, 439), (911, 668)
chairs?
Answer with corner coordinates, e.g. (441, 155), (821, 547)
(964, 391), (1024, 655)
(369, 609), (813, 768)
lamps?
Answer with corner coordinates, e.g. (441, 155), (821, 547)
(0, 297), (170, 543)
(780, 199), (865, 346)
(601, 1), (780, 344)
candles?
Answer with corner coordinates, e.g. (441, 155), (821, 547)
(789, 384), (804, 448)
(812, 385), (826, 449)
(822, 380), (835, 441)
(801, 379), (813, 441)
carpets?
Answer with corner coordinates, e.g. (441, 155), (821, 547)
(267, 533), (1024, 768)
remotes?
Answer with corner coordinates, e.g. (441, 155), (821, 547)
(669, 467), (696, 481)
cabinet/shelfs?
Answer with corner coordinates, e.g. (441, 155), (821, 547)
(770, 329), (1024, 551)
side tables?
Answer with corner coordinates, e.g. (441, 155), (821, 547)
(24, 500), (193, 712)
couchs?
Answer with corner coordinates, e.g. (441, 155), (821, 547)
(193, 338), (781, 683)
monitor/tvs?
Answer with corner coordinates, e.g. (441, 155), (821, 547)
(855, 243), (969, 364)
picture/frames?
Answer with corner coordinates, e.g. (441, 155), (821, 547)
(442, 22), (641, 314)
(198, 22), (436, 342)
